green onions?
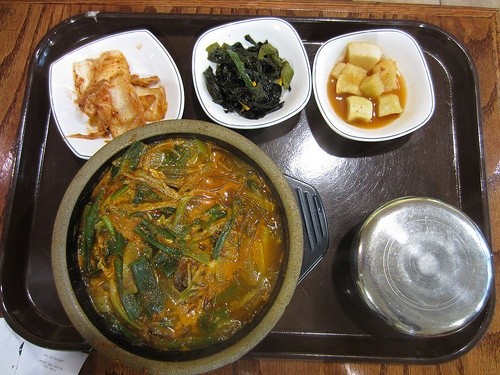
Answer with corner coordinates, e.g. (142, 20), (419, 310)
(73, 137), (278, 354)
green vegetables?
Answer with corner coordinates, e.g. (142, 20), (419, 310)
(203, 34), (294, 119)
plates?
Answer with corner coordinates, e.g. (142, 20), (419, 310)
(313, 27), (436, 142)
(190, 16), (312, 128)
(47, 29), (185, 160)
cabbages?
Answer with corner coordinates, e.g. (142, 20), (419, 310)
(66, 50), (167, 144)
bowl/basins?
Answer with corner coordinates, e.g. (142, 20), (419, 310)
(49, 118), (305, 374)
(342, 194), (494, 339)
